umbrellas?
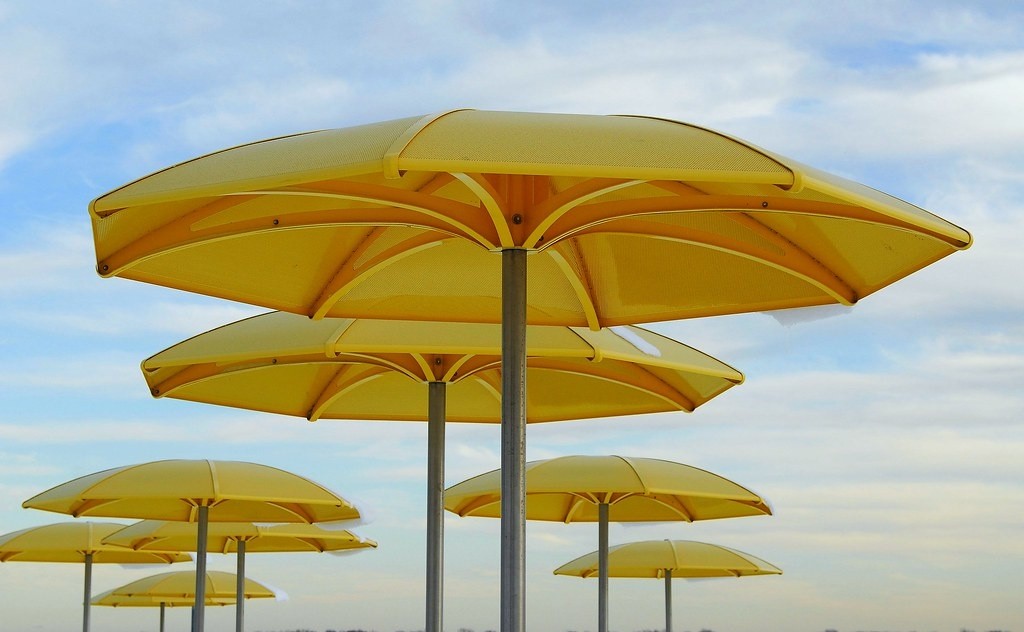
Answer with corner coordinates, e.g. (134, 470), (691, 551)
(23, 460), (361, 632)
(88, 105), (972, 632)
(83, 586), (239, 632)
(115, 571), (276, 632)
(0, 520), (193, 632)
(553, 539), (783, 632)
(442, 453), (776, 632)
(101, 517), (378, 632)
